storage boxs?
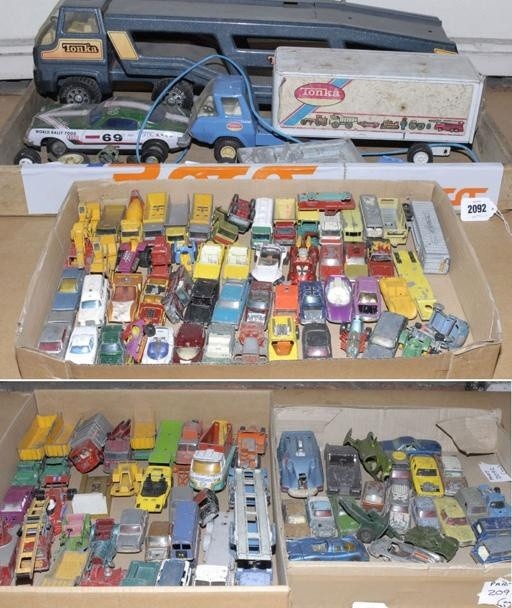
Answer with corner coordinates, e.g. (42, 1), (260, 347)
(2, 76), (511, 222)
(0, 377), (291, 608)
(13, 179), (505, 378)
(270, 404), (511, 606)
